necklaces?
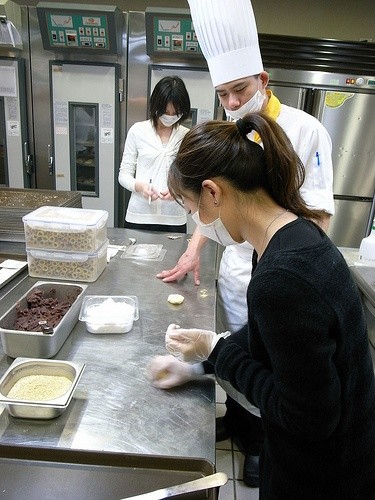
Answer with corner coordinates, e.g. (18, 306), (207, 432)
(256, 207), (295, 263)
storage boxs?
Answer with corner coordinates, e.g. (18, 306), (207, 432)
(79, 295), (139, 334)
(23, 205), (109, 283)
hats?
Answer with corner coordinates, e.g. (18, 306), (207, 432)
(187, 0), (264, 88)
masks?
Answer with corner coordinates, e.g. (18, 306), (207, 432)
(157, 111), (183, 128)
(191, 188), (246, 246)
(225, 77), (266, 120)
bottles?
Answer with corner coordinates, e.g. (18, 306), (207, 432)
(359, 219), (375, 264)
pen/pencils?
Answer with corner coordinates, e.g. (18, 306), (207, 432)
(148, 178), (153, 204)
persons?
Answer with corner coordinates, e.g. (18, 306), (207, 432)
(142, 111), (375, 499)
(154, 0), (336, 490)
(118, 74), (192, 235)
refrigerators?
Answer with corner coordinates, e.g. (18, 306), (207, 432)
(223, 32), (375, 249)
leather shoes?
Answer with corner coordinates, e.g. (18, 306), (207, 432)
(243, 455), (259, 488)
(216, 416), (229, 442)
